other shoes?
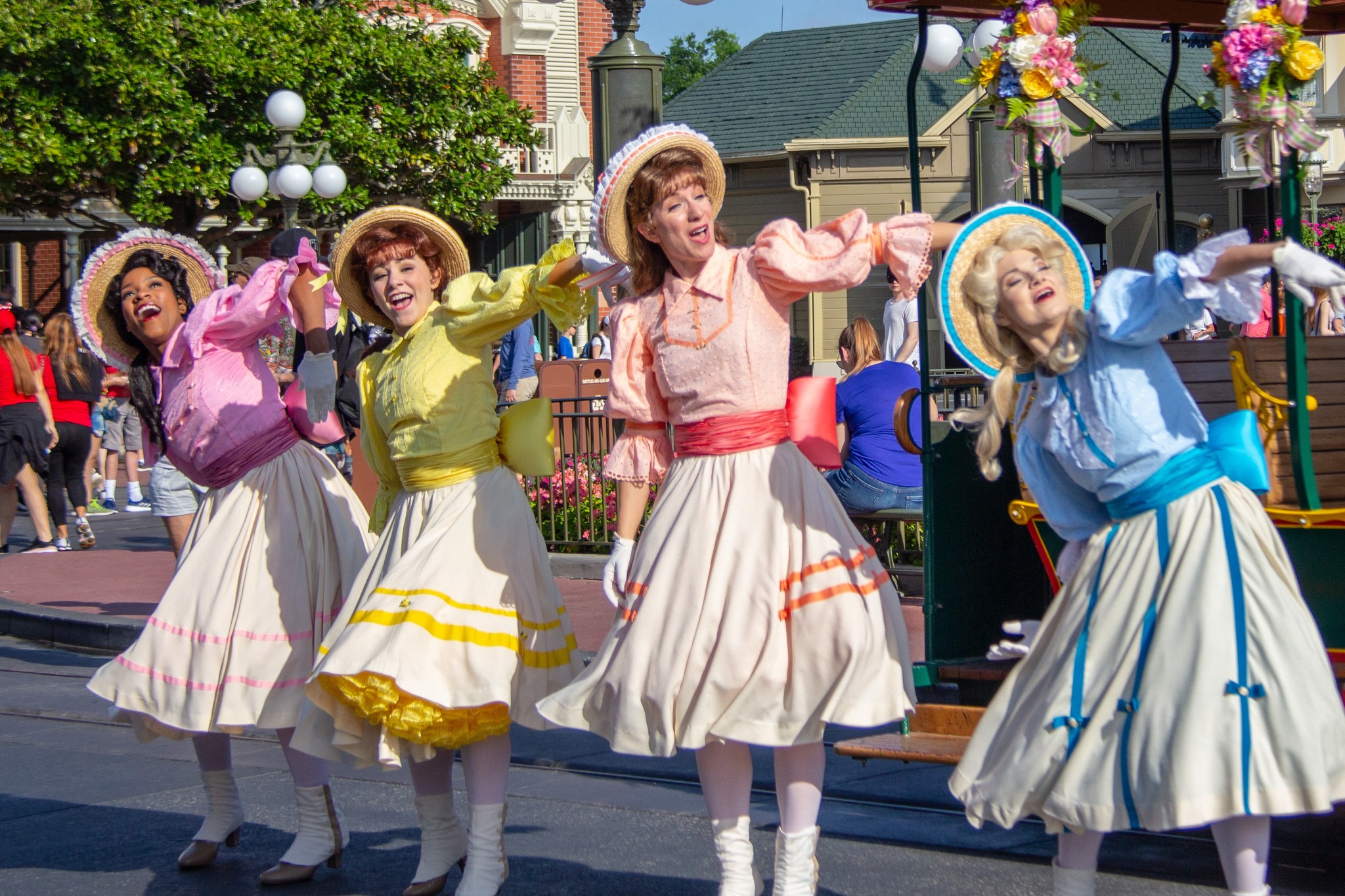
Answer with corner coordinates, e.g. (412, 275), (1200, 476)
(138, 461), (153, 471)
(20, 538), (58, 554)
(52, 536), (72, 551)
(74, 517), (96, 550)
(0, 543), (10, 554)
(98, 491), (107, 499)
(91, 471), (104, 490)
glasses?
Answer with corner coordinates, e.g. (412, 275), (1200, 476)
(229, 271), (238, 280)
(885, 275), (899, 284)
(570, 326), (578, 330)
(836, 351), (845, 369)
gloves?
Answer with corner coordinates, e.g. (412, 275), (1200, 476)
(985, 619), (1043, 663)
(296, 346), (338, 425)
(1270, 235), (1345, 311)
(580, 249), (637, 308)
(603, 532), (637, 611)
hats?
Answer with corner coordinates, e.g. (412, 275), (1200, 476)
(223, 257), (269, 276)
(590, 122), (728, 264)
(603, 315), (610, 327)
(329, 204), (473, 332)
(938, 202), (1098, 389)
(72, 225), (228, 371)
(564, 321), (579, 331)
(271, 226), (329, 263)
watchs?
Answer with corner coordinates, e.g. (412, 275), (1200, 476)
(1201, 329), (1205, 334)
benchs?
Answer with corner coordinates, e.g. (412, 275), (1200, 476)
(847, 508), (924, 601)
(997, 338), (1239, 525)
(1227, 333), (1345, 530)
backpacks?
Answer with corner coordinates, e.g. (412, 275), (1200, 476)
(582, 332), (605, 362)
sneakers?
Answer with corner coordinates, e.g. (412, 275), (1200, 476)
(102, 500), (118, 514)
(124, 496), (151, 512)
(86, 498), (114, 516)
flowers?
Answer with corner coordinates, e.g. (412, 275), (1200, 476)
(1197, 0), (1327, 180)
(957, 0), (1123, 136)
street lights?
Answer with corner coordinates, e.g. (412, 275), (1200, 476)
(911, 13), (1027, 211)
(230, 88), (348, 229)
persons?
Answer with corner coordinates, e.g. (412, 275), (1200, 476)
(1089, 259), (1107, 294)
(69, 226), (377, 886)
(819, 317), (940, 536)
(558, 322), (577, 359)
(938, 200), (1345, 896)
(303, 206), (584, 896)
(535, 124), (963, 896)
(591, 315), (612, 360)
(1159, 275), (1345, 340)
(883, 262), (921, 375)
(492, 317), (544, 479)
(0, 227), (393, 555)
